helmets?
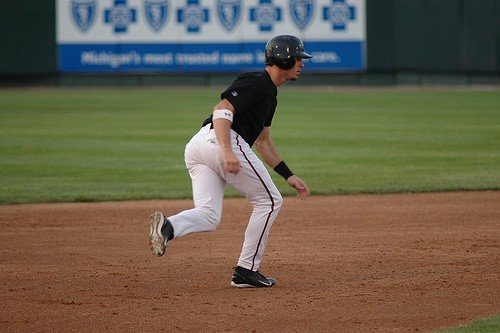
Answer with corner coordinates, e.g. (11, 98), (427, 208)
(265, 35), (313, 70)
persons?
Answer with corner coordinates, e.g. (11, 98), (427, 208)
(146, 35), (314, 288)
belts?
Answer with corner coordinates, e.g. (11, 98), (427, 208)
(210, 123), (213, 129)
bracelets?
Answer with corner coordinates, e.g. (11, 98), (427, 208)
(274, 161), (294, 181)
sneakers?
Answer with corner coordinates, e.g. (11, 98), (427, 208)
(147, 211), (170, 257)
(230, 266), (275, 287)
